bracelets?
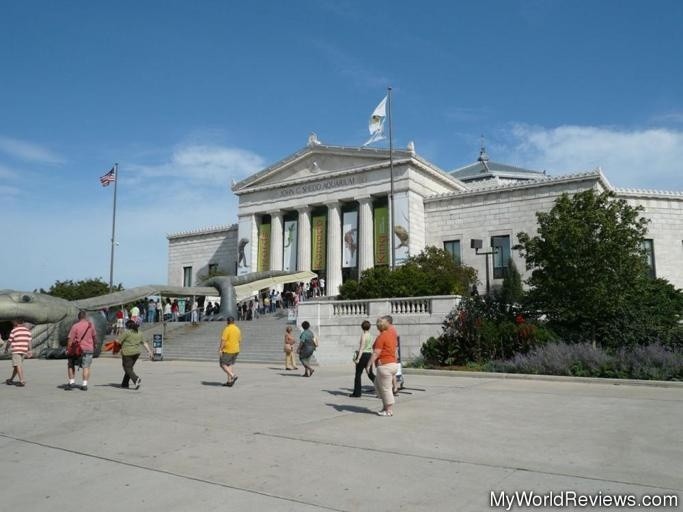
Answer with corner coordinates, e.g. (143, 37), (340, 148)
(218, 350), (222, 353)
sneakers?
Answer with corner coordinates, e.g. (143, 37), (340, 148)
(136, 378), (141, 389)
(378, 411), (393, 416)
(80, 386), (87, 390)
(65, 383), (74, 390)
(7, 380), (24, 386)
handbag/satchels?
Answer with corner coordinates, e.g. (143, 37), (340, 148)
(70, 342), (81, 356)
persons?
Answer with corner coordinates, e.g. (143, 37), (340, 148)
(100, 270), (327, 335)
(2, 319), (33, 387)
(217, 316), (242, 388)
(365, 317), (398, 418)
(382, 314), (402, 395)
(349, 319), (377, 397)
(284, 326), (298, 369)
(115, 319), (153, 390)
(62, 309), (97, 391)
(295, 321), (317, 377)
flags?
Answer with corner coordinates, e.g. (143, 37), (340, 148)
(97, 166), (115, 188)
(361, 94), (388, 148)
